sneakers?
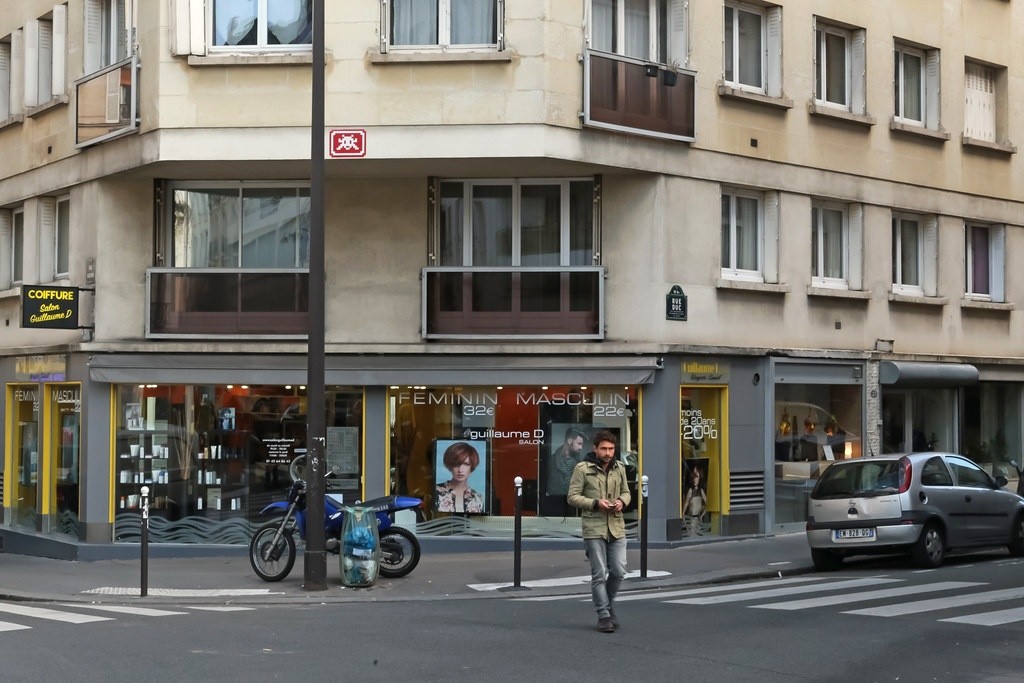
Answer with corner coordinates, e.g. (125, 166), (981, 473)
(608, 614), (620, 629)
(596, 617), (614, 632)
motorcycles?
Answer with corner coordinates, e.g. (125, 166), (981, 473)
(245, 449), (426, 580)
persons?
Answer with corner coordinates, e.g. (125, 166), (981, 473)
(436, 442), (485, 512)
(253, 398), (273, 413)
(566, 430), (632, 633)
(220, 409), (234, 429)
(547, 427), (589, 494)
(683, 464), (708, 536)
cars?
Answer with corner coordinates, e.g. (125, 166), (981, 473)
(807, 451), (1023, 568)
